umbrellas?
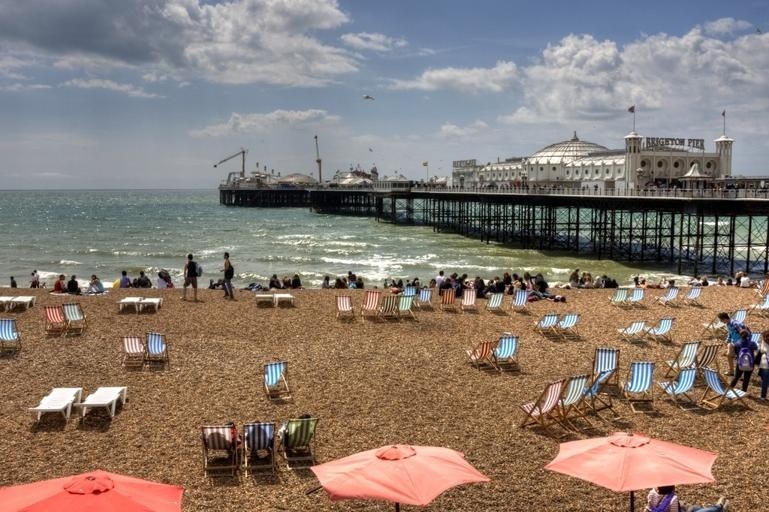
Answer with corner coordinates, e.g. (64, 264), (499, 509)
(541, 428), (720, 512)
(0, 470), (186, 512)
(309, 443), (493, 512)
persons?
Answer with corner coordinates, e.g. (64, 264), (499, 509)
(179, 253), (198, 301)
(593, 183), (599, 195)
(686, 270), (758, 288)
(633, 275), (679, 290)
(718, 310), (769, 403)
(207, 413), (312, 451)
(487, 182), (565, 194)
(321, 276), (331, 289)
(644, 486), (729, 512)
(219, 251), (234, 298)
(268, 273), (305, 289)
(10, 269), (174, 295)
(333, 270), (364, 289)
(568, 268), (618, 289)
(719, 181), (745, 198)
(384, 269), (566, 303)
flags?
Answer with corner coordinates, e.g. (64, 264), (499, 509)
(722, 111), (725, 116)
(627, 105), (635, 114)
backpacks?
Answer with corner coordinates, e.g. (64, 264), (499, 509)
(736, 347), (754, 372)
(195, 263), (202, 277)
(740, 325), (751, 339)
(225, 264), (234, 280)
